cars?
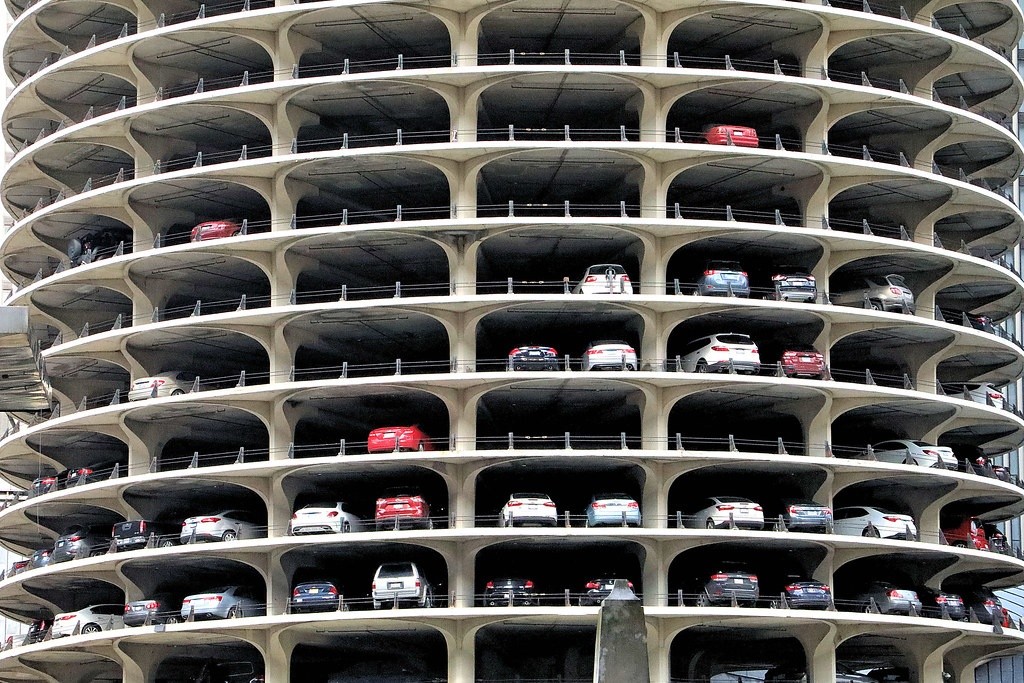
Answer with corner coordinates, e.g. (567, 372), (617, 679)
(0, 561), (1010, 683)
(686, 495), (765, 529)
(678, 332), (760, 375)
(573, 262), (634, 294)
(943, 514), (1012, 554)
(189, 215), (240, 242)
(951, 442), (1024, 489)
(779, 497), (830, 533)
(775, 342), (826, 380)
(499, 492), (558, 528)
(1, 489), (435, 581)
(834, 507), (917, 540)
(583, 339), (638, 371)
(698, 122), (759, 147)
(366, 425), (438, 452)
(941, 307), (995, 334)
(938, 381), (1006, 410)
(26, 459), (124, 496)
(849, 438), (959, 471)
(831, 274), (915, 312)
(127, 367), (221, 401)
(505, 342), (560, 371)
(584, 492), (642, 526)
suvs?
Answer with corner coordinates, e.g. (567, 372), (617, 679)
(692, 260), (750, 297)
(68, 227), (132, 261)
(769, 264), (817, 303)
